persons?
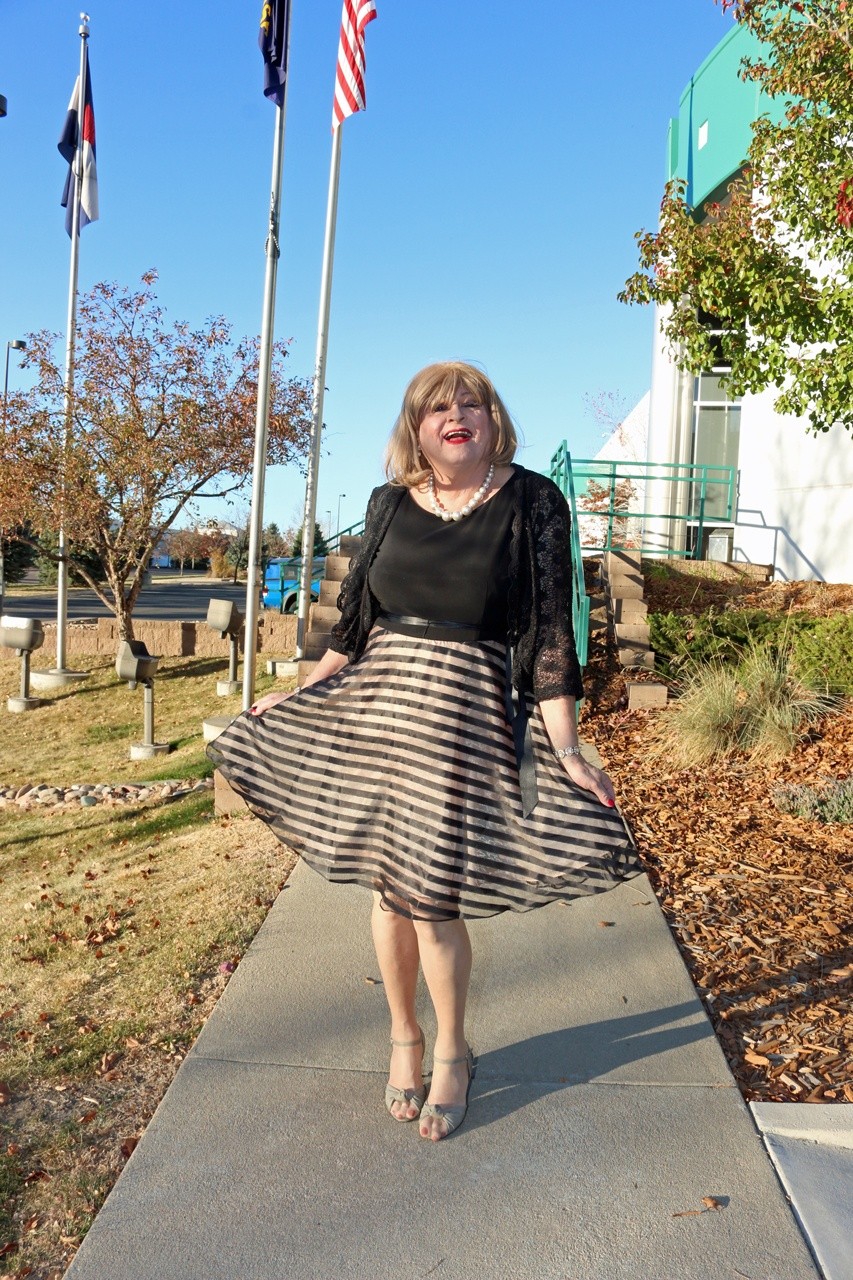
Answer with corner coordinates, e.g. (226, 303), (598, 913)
(205, 359), (647, 1140)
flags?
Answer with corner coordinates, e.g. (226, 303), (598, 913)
(57, 44), (100, 240)
(258, 0), (291, 109)
(330, 0), (377, 134)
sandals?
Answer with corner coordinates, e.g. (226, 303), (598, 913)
(385, 1025), (426, 1121)
(419, 1039), (474, 1141)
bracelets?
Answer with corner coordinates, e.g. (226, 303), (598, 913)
(553, 746), (580, 758)
(295, 686), (301, 694)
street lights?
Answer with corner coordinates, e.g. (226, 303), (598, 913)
(336, 494), (346, 556)
(326, 510), (330, 542)
(2, 340), (27, 438)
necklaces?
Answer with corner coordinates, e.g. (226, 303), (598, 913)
(428, 464), (494, 521)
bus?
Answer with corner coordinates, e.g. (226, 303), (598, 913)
(259, 555), (329, 617)
